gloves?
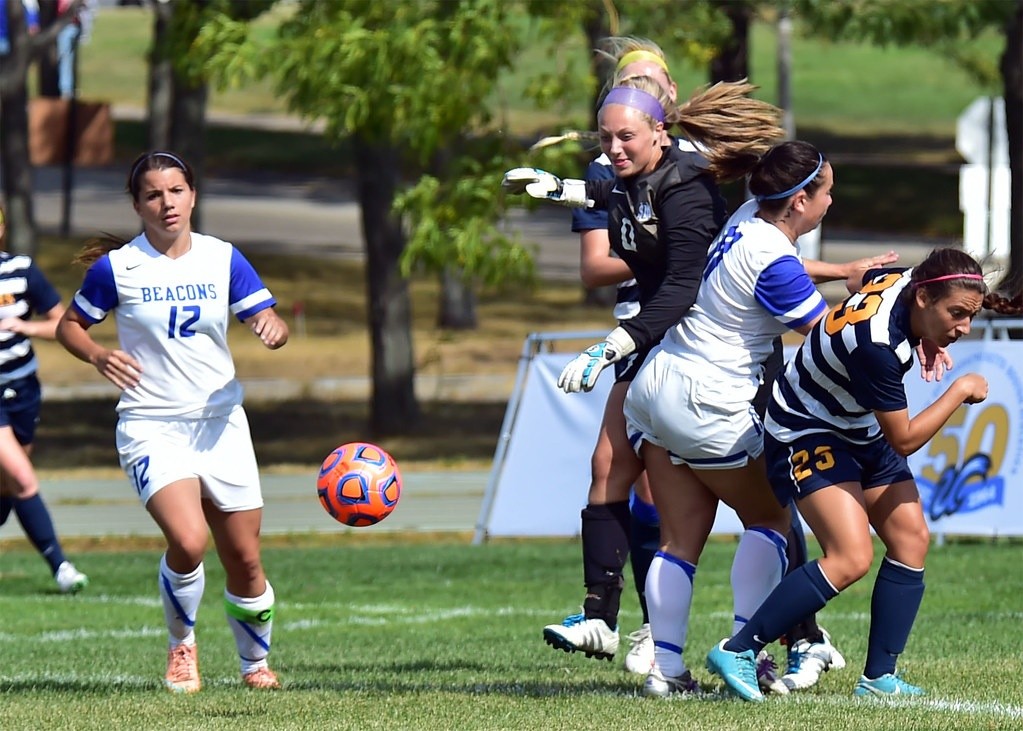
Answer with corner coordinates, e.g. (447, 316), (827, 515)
(501, 168), (587, 209)
(558, 325), (636, 393)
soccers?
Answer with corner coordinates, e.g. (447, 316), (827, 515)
(316, 441), (401, 528)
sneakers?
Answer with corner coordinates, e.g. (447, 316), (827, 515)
(625, 623), (655, 674)
(854, 669), (926, 696)
(781, 634), (833, 690)
(643, 669), (703, 698)
(542, 606), (620, 661)
(755, 650), (790, 695)
(164, 644), (201, 694)
(55, 561), (87, 592)
(817, 624), (846, 670)
(704, 638), (764, 703)
(243, 667), (280, 689)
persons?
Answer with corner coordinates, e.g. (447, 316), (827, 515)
(0, 183), (91, 595)
(501, 79), (849, 697)
(620, 139), (901, 708)
(565, 31), (813, 685)
(56, 150), (292, 699)
(701, 244), (1020, 700)
